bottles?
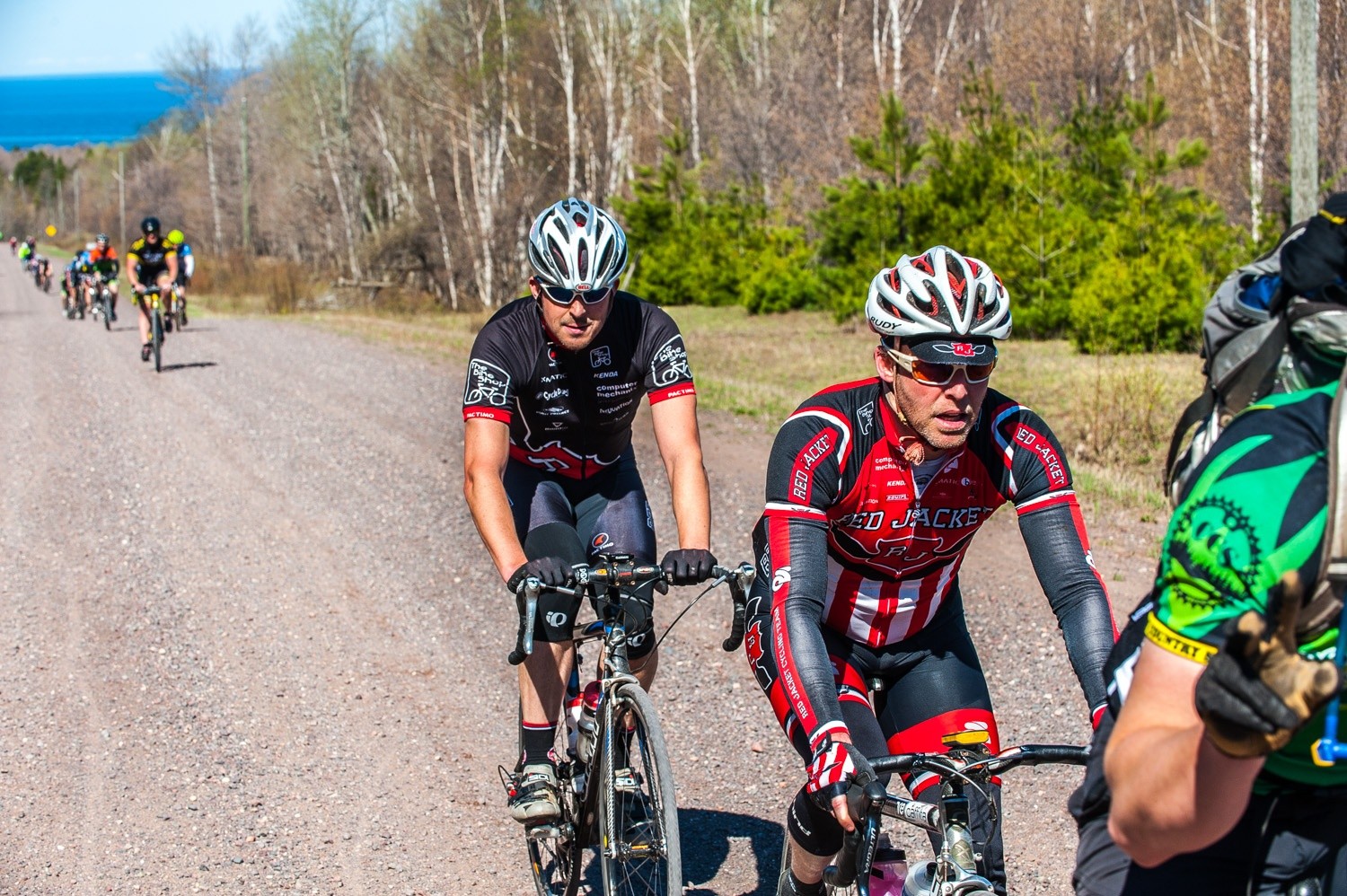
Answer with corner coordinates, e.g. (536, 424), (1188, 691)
(565, 691), (584, 755)
(577, 679), (602, 763)
(901, 860), (939, 896)
(868, 832), (909, 896)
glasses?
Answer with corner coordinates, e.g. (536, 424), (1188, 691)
(534, 275), (613, 304)
(880, 338), (998, 386)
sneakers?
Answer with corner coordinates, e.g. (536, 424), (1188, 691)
(142, 345), (150, 361)
(508, 764), (561, 824)
(615, 767), (642, 794)
(165, 312), (172, 332)
(110, 311), (117, 321)
(777, 868), (827, 896)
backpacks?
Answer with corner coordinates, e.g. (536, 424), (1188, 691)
(1164, 193), (1347, 507)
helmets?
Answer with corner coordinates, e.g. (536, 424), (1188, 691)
(96, 233), (109, 243)
(528, 197), (628, 292)
(168, 229), (184, 244)
(865, 244), (1013, 340)
(140, 216), (161, 233)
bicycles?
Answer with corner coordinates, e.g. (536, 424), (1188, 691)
(779, 728), (1090, 896)
(75, 273), (182, 374)
(507, 552), (757, 896)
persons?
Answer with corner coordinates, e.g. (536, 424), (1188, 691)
(742, 243), (1120, 896)
(1067, 379), (1347, 896)
(8, 216), (197, 363)
(459, 198), (719, 855)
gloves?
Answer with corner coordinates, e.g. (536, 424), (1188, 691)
(653, 548), (718, 596)
(507, 557), (575, 593)
(1196, 572), (1338, 759)
